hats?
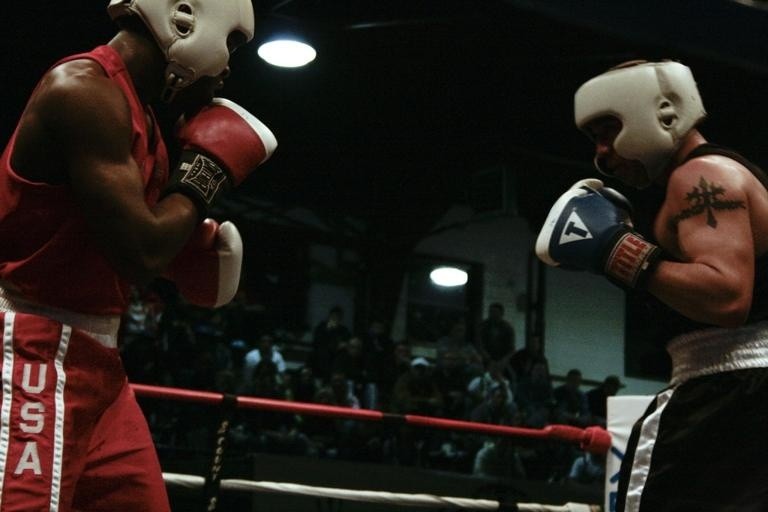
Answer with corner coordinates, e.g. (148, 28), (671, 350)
(409, 356), (430, 369)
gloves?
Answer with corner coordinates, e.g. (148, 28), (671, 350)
(155, 95), (281, 223)
(157, 211), (246, 308)
(532, 174), (665, 291)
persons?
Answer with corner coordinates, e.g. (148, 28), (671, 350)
(535, 58), (768, 512)
(0, 1), (280, 512)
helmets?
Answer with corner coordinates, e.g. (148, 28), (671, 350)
(565, 57), (708, 192)
(105, 0), (259, 98)
